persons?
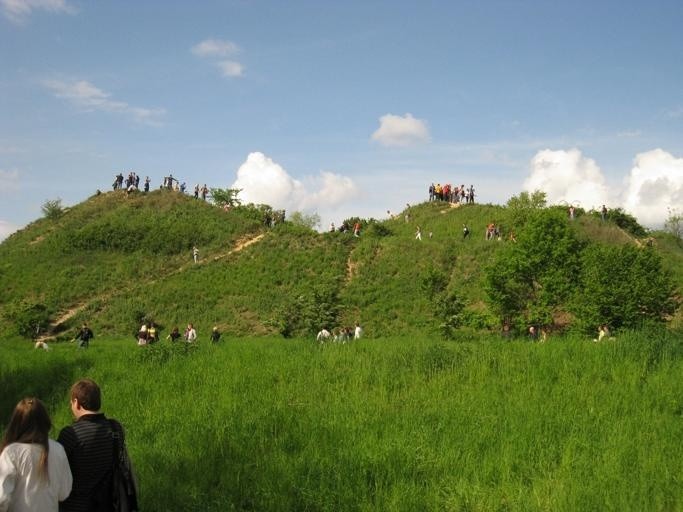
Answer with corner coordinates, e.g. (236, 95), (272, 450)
(134, 319), (221, 347)
(604, 325), (610, 337)
(329, 180), (517, 245)
(316, 321), (362, 343)
(192, 246), (198, 264)
(54, 379), (125, 511)
(593, 325), (606, 343)
(569, 204), (576, 219)
(94, 172), (210, 201)
(34, 341), (49, 352)
(540, 329), (548, 342)
(0, 399), (74, 511)
(261, 208), (286, 229)
(600, 204), (607, 225)
(223, 201), (230, 215)
(501, 326), (511, 338)
(529, 327), (538, 340)
(70, 323), (95, 350)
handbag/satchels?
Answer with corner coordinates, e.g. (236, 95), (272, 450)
(110, 418), (138, 511)
